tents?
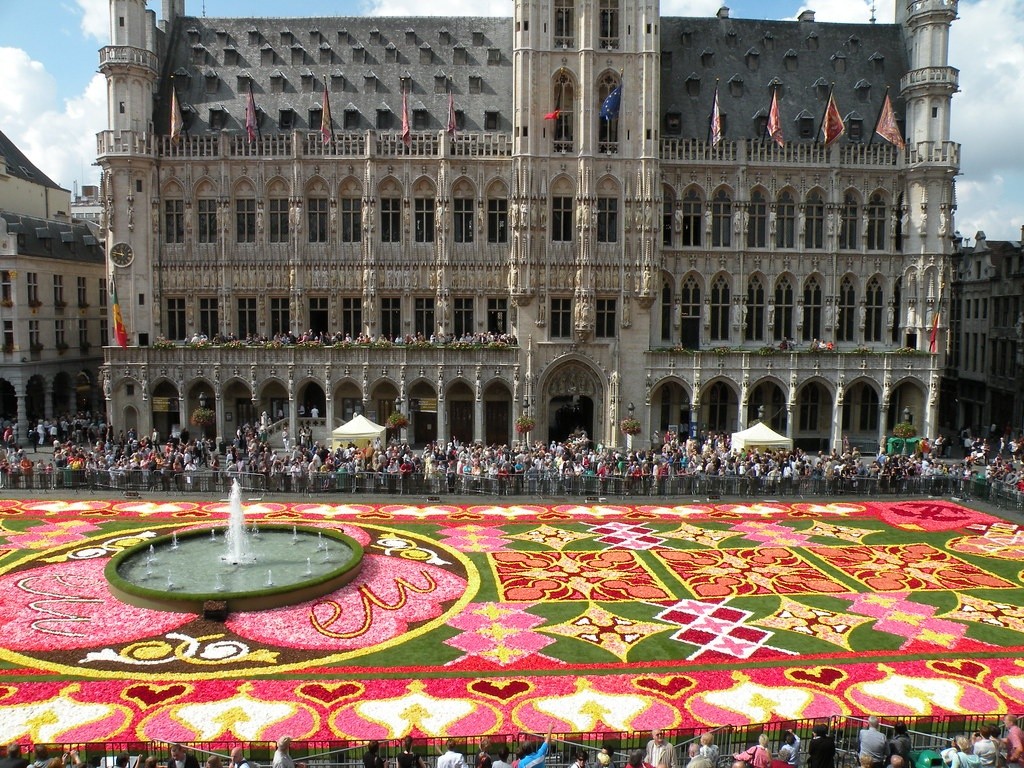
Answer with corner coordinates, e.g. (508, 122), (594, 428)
(331, 414), (386, 453)
(731, 422), (793, 454)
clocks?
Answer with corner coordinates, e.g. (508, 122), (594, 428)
(108, 242), (134, 267)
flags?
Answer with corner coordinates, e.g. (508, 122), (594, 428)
(402, 91), (411, 148)
(709, 88), (722, 147)
(170, 88), (184, 145)
(447, 93), (457, 142)
(599, 83), (621, 119)
(768, 91), (784, 148)
(322, 90), (331, 144)
(246, 91), (258, 143)
(821, 93), (845, 146)
(876, 96), (903, 150)
(930, 301), (941, 352)
(544, 76), (563, 120)
(113, 286), (127, 350)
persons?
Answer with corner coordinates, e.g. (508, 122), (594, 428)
(811, 338), (833, 350)
(191, 331), (208, 344)
(0, 404), (1024, 510)
(212, 332), (237, 344)
(780, 337), (797, 350)
(246, 329), (517, 344)
(0, 714), (1024, 768)
